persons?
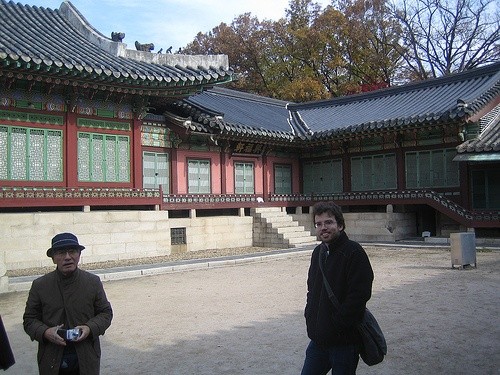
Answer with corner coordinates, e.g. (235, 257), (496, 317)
(0, 314), (16, 371)
(300, 203), (375, 375)
(22, 233), (113, 375)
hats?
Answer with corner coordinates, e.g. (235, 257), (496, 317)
(47, 233), (85, 257)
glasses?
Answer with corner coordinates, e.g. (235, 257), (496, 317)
(314, 221), (338, 228)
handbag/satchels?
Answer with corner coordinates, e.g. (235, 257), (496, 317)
(350, 307), (387, 366)
